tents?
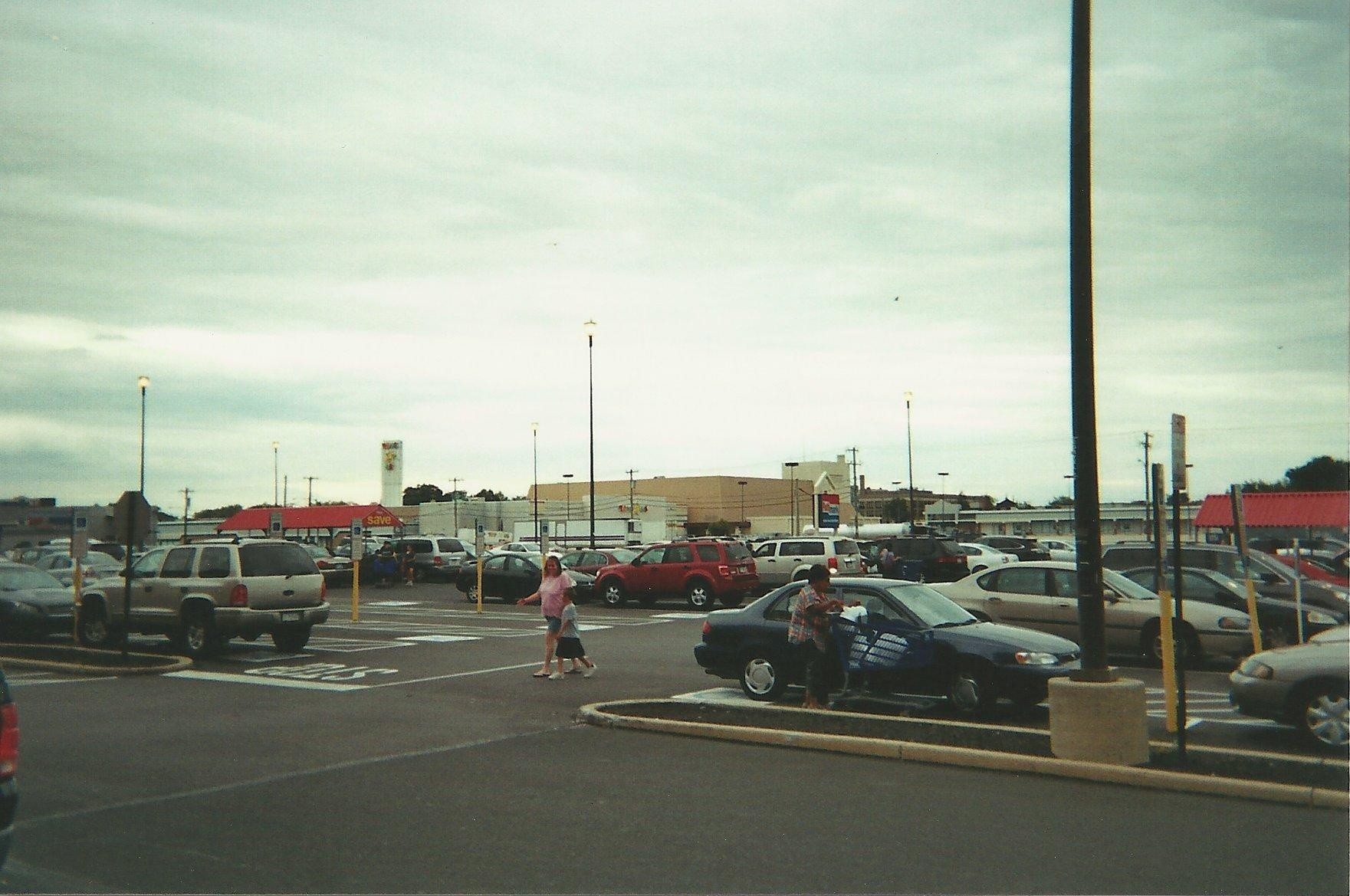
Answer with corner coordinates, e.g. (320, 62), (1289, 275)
(214, 504), (406, 585)
(1192, 489), (1350, 529)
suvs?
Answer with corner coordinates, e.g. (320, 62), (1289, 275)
(1099, 540), (1350, 621)
(746, 537), (862, 594)
(597, 539), (758, 611)
(972, 535), (1051, 562)
(79, 538), (331, 653)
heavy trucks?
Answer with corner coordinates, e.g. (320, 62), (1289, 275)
(804, 523), (941, 538)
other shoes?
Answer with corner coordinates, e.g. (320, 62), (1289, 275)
(405, 580), (413, 586)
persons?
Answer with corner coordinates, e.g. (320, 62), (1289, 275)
(517, 556), (582, 677)
(787, 563), (841, 711)
(882, 552), (899, 570)
(548, 587), (597, 680)
(877, 549), (888, 563)
(376, 541), (417, 586)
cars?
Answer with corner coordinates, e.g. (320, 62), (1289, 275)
(1034, 540), (1077, 562)
(455, 553), (596, 605)
(28, 551), (125, 587)
(694, 576), (1082, 715)
(1230, 639), (1349, 752)
(956, 542), (1019, 575)
(557, 548), (644, 576)
(457, 546), (495, 560)
(1108, 565), (1349, 647)
(488, 541), (562, 561)
(0, 563), (75, 640)
(1240, 554), (1349, 590)
(914, 560), (1261, 665)
(297, 547), (354, 585)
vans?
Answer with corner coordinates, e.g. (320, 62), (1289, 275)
(872, 534), (970, 585)
(18, 542), (127, 574)
(378, 535), (467, 581)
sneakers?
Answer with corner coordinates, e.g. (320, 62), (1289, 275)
(584, 663), (597, 678)
(531, 669), (551, 676)
(565, 665), (583, 672)
(549, 671), (565, 679)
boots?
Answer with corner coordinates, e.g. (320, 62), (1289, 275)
(810, 697), (827, 709)
(803, 690), (813, 707)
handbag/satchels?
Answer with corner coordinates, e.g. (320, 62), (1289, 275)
(883, 560), (892, 570)
